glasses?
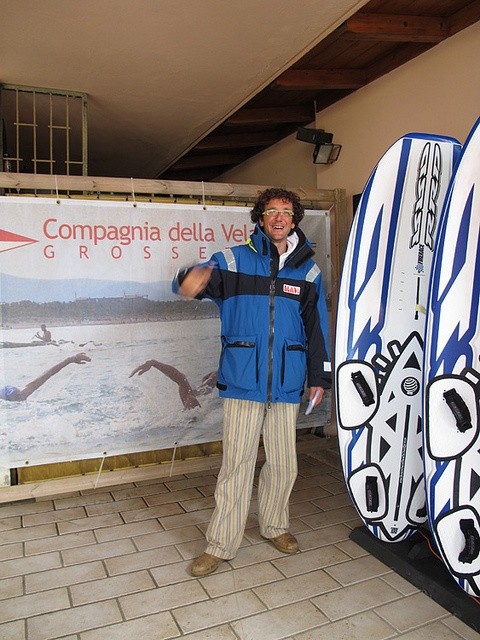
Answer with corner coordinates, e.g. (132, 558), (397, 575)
(261, 209), (294, 216)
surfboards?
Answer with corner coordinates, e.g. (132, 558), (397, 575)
(335, 131), (466, 546)
(421, 115), (480, 600)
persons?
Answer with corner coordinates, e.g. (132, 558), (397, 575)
(171, 188), (331, 575)
(32, 323), (52, 348)
(0, 352), (92, 402)
(128, 359), (218, 410)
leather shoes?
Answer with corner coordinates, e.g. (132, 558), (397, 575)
(261, 533), (299, 553)
(191, 552), (225, 576)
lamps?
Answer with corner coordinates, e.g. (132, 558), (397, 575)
(296, 126), (342, 165)
